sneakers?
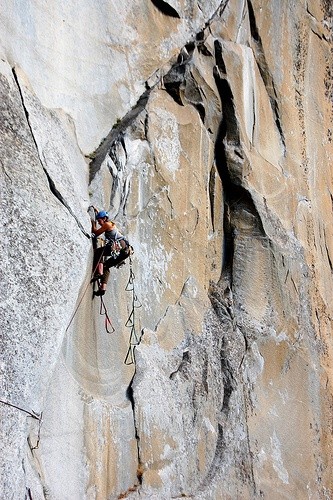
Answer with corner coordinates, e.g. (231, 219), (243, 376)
(95, 288), (105, 296)
(91, 272), (102, 282)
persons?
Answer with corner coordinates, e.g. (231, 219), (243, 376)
(88, 205), (131, 296)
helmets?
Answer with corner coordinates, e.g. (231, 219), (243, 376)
(96, 210), (109, 220)
